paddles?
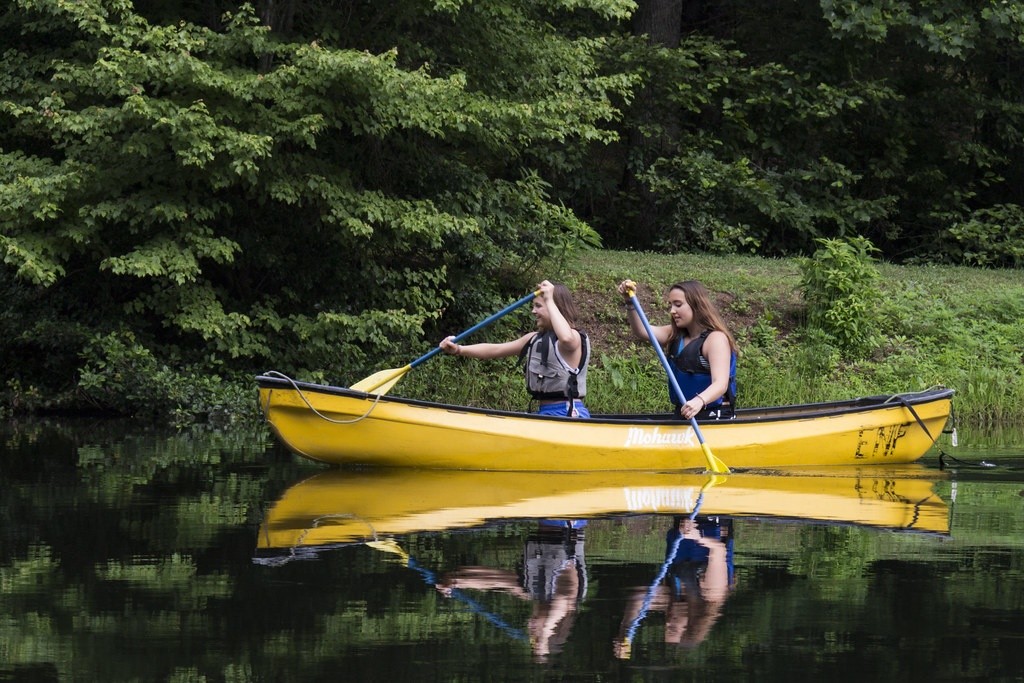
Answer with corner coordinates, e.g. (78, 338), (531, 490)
(624, 282), (731, 475)
(366, 538), (537, 649)
(622, 476), (728, 658)
(349, 289), (542, 396)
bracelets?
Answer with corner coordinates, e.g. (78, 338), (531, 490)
(696, 392), (706, 406)
(456, 345), (461, 355)
(625, 299), (636, 310)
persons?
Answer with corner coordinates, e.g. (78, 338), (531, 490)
(618, 277), (739, 420)
(613, 516), (737, 659)
(440, 280), (592, 419)
(436, 519), (588, 665)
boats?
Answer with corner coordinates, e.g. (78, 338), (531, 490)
(255, 374), (956, 473)
(255, 474), (953, 564)
